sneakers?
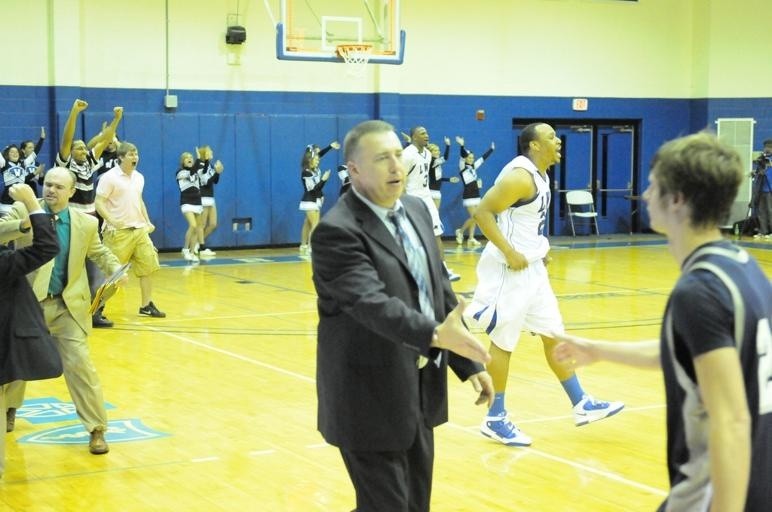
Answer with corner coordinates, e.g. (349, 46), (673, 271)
(479, 410), (533, 446)
(455, 228), (464, 245)
(91, 308), (113, 327)
(139, 302), (166, 318)
(467, 237), (482, 248)
(200, 248), (216, 256)
(194, 244), (199, 254)
(572, 392), (625, 428)
(181, 248), (189, 257)
(185, 252), (199, 262)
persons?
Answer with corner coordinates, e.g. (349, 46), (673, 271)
(2, 99), (167, 453)
(310, 118), (495, 511)
(398, 121), (463, 284)
(550, 131), (771, 511)
(175, 145), (210, 264)
(297, 149), (333, 254)
(192, 142), (224, 258)
(426, 133), (461, 210)
(400, 130), (412, 149)
(305, 138), (341, 159)
(453, 134), (495, 246)
(465, 120), (628, 448)
(751, 137), (771, 238)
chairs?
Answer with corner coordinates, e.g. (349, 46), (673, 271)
(565, 189), (600, 238)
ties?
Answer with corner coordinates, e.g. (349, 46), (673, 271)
(391, 213), (437, 326)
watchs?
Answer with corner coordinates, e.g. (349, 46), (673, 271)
(431, 326), (440, 349)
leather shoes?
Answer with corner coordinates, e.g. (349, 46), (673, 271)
(6, 408), (15, 432)
(89, 429), (109, 454)
